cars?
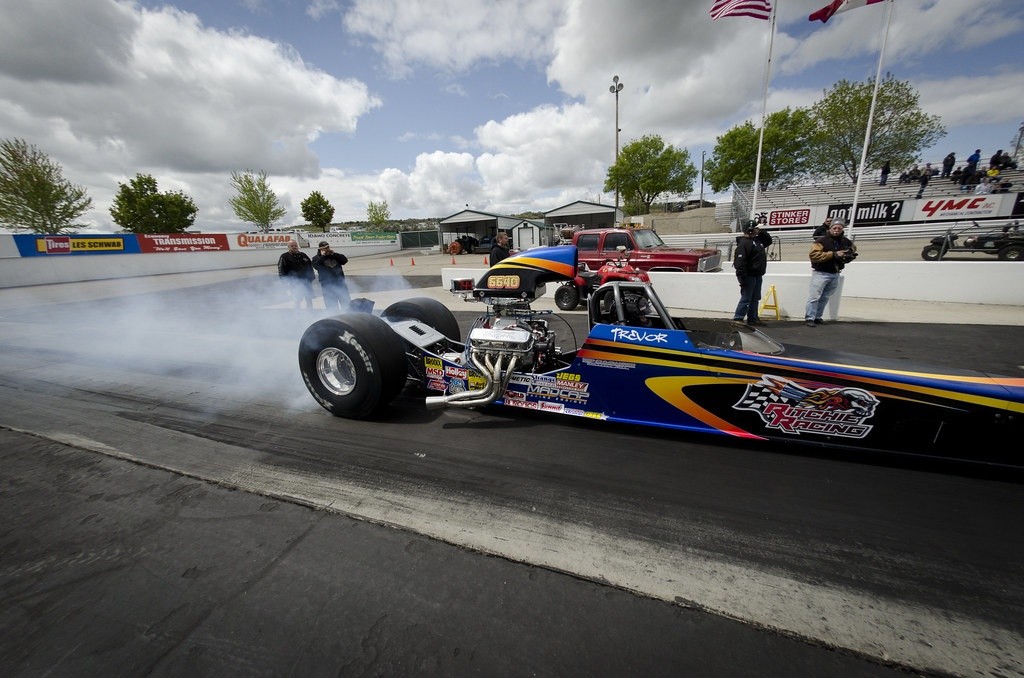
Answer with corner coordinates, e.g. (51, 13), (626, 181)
(571, 228), (720, 272)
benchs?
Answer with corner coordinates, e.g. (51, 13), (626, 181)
(740, 169), (1024, 210)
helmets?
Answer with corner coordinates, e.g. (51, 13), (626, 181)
(610, 295), (652, 329)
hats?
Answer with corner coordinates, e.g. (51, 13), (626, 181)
(741, 219), (764, 231)
(319, 241), (329, 248)
(830, 217), (845, 228)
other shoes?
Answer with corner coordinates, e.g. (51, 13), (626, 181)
(733, 319), (746, 326)
(747, 319), (768, 325)
(806, 321), (816, 327)
(814, 319), (827, 324)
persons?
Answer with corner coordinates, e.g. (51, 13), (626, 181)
(312, 241), (352, 314)
(812, 217), (833, 240)
(899, 149), (1017, 199)
(804, 217), (858, 327)
(278, 240), (315, 309)
(489, 232), (511, 268)
(733, 220), (772, 326)
(879, 160), (891, 185)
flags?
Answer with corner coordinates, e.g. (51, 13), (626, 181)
(710, 0), (773, 22)
(809, 0), (887, 23)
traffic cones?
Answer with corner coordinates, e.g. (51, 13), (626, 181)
(412, 258), (415, 266)
(483, 256), (488, 265)
(390, 258), (393, 266)
(452, 258), (455, 265)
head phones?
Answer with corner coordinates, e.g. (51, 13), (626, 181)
(741, 221), (750, 234)
